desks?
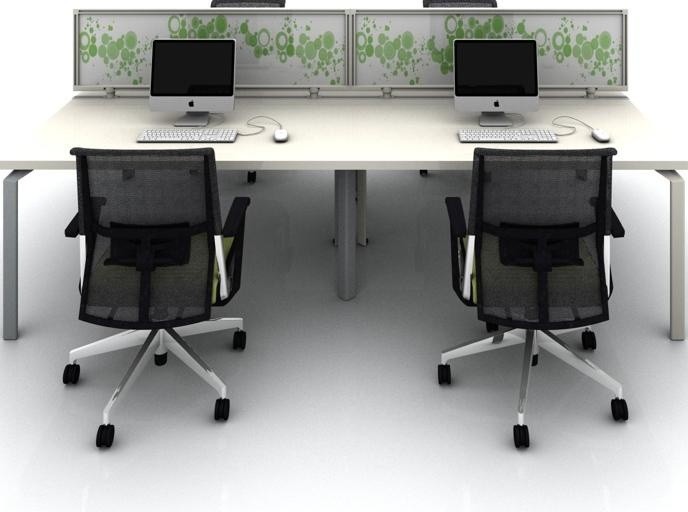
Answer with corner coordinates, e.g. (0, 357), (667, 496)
(0, 95), (688, 342)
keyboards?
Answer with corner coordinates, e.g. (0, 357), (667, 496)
(136, 126), (238, 143)
(459, 127), (558, 143)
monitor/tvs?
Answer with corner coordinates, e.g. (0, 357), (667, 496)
(452, 38), (540, 127)
(149, 38), (237, 127)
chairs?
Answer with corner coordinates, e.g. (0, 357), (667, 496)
(62, 147), (252, 446)
(436, 148), (630, 449)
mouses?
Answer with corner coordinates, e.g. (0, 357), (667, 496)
(592, 127), (610, 142)
(274, 128), (288, 142)
